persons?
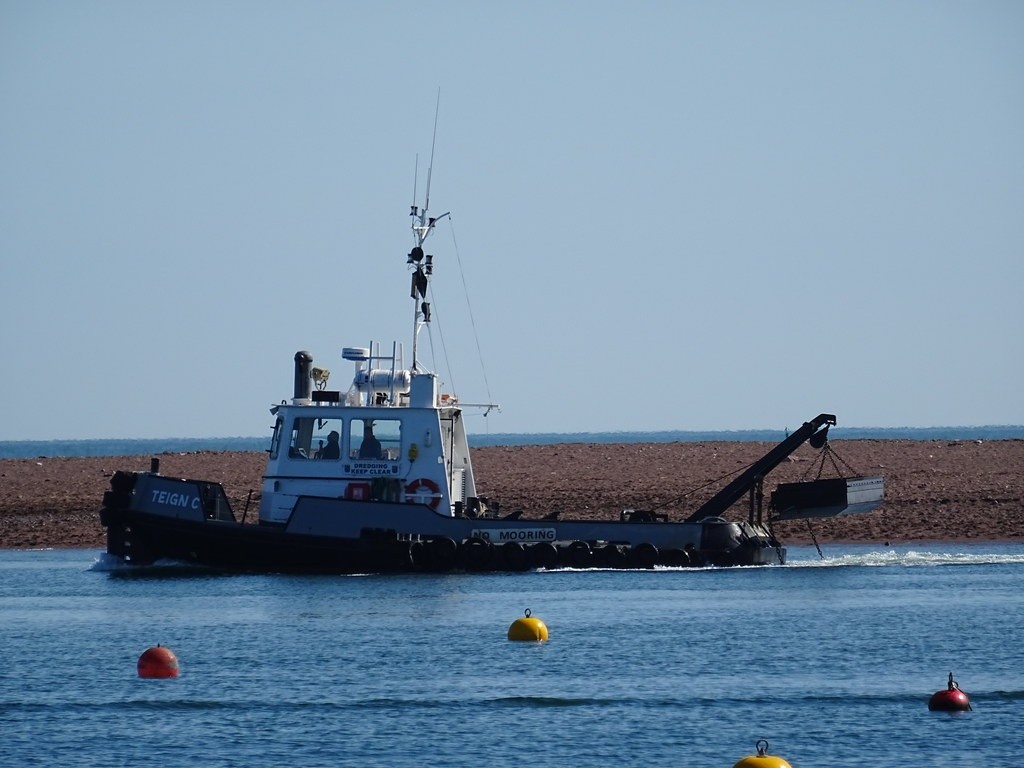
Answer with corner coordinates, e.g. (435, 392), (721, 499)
(318, 430), (340, 459)
(359, 426), (382, 460)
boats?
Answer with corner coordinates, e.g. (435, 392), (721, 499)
(100, 86), (884, 578)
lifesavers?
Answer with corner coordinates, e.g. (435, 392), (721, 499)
(403, 539), (425, 574)
(404, 476), (441, 510)
(429, 535), (457, 552)
(530, 541), (557, 559)
(663, 548), (691, 565)
(462, 536), (492, 553)
(498, 539), (525, 560)
(631, 540), (659, 559)
(568, 540), (591, 556)
